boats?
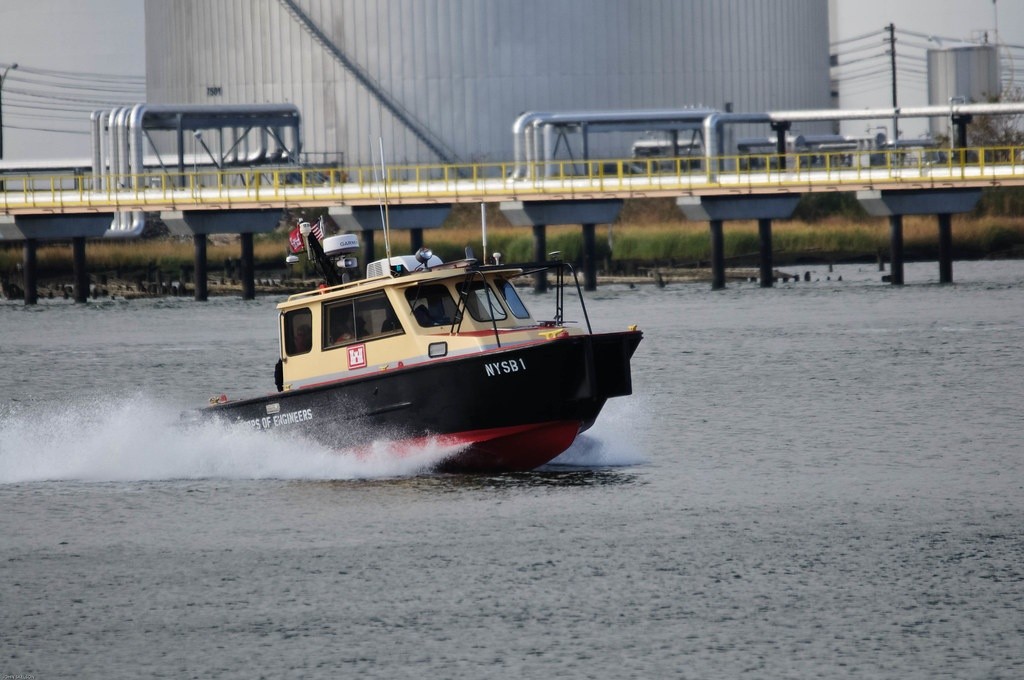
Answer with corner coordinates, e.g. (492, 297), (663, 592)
(184, 240), (644, 478)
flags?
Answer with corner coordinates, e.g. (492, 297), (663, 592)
(288, 225), (305, 253)
(311, 217), (324, 241)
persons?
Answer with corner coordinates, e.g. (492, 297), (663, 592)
(415, 300), (454, 327)
(294, 325), (311, 353)
(330, 297), (400, 344)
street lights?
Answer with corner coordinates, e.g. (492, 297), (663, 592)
(1, 63), (21, 163)
